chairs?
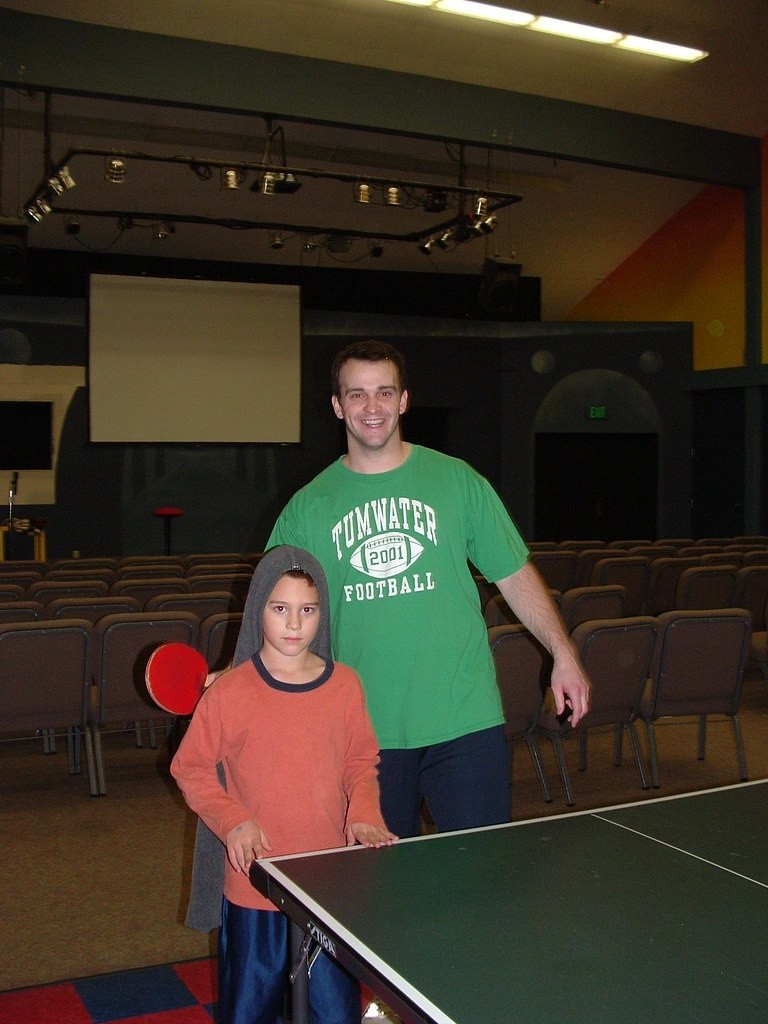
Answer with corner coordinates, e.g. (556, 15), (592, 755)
(0, 535), (768, 807)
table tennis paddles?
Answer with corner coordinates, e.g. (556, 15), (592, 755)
(145, 641), (209, 715)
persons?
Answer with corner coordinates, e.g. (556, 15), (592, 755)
(263, 340), (593, 841)
(170, 545), (400, 1024)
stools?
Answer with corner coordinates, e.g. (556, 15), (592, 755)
(152, 506), (184, 555)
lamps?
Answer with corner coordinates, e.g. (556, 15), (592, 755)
(23, 154), (498, 257)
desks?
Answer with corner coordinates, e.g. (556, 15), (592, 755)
(250, 774), (768, 1024)
